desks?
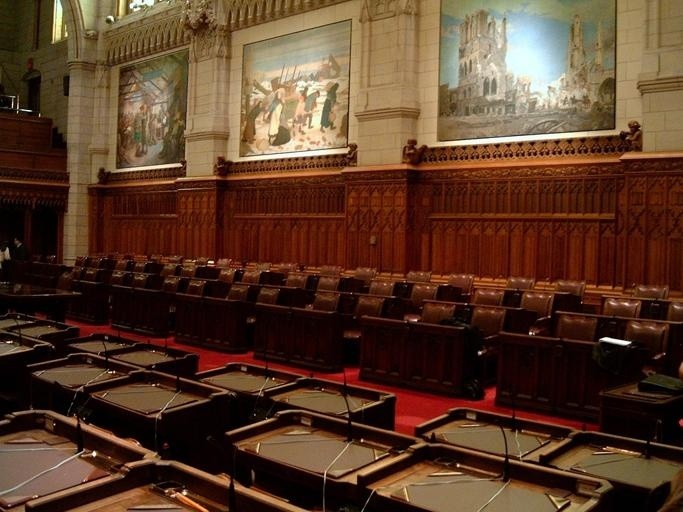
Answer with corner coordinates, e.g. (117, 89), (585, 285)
(598, 384), (682, 443)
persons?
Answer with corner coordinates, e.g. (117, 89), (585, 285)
(117, 103), (185, 167)
(240, 69), (341, 148)
(11, 234), (30, 262)
(0, 240), (10, 264)
